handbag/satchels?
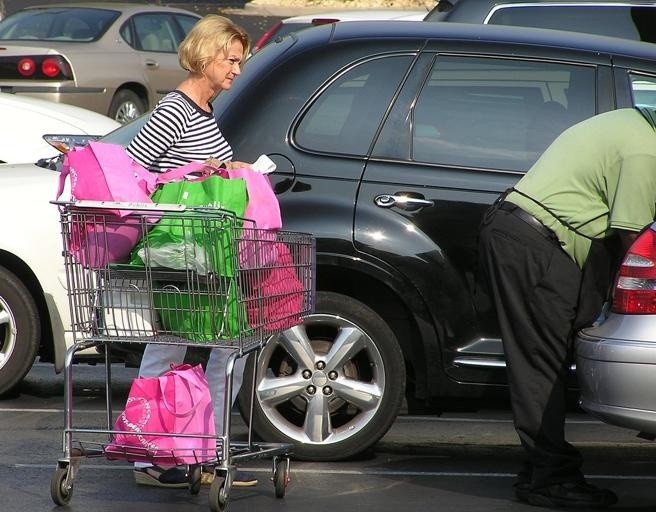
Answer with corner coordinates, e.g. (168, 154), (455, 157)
(105, 362), (216, 464)
(58, 141), (305, 338)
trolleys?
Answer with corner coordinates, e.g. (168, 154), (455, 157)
(49, 191), (319, 512)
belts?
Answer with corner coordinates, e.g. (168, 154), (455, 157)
(497, 200), (554, 239)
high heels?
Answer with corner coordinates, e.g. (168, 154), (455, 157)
(200, 462), (258, 487)
(134, 461), (189, 488)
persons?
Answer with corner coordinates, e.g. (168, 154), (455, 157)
(480, 103), (656, 512)
(124, 13), (257, 487)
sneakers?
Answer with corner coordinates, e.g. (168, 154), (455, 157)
(512, 478), (618, 509)
